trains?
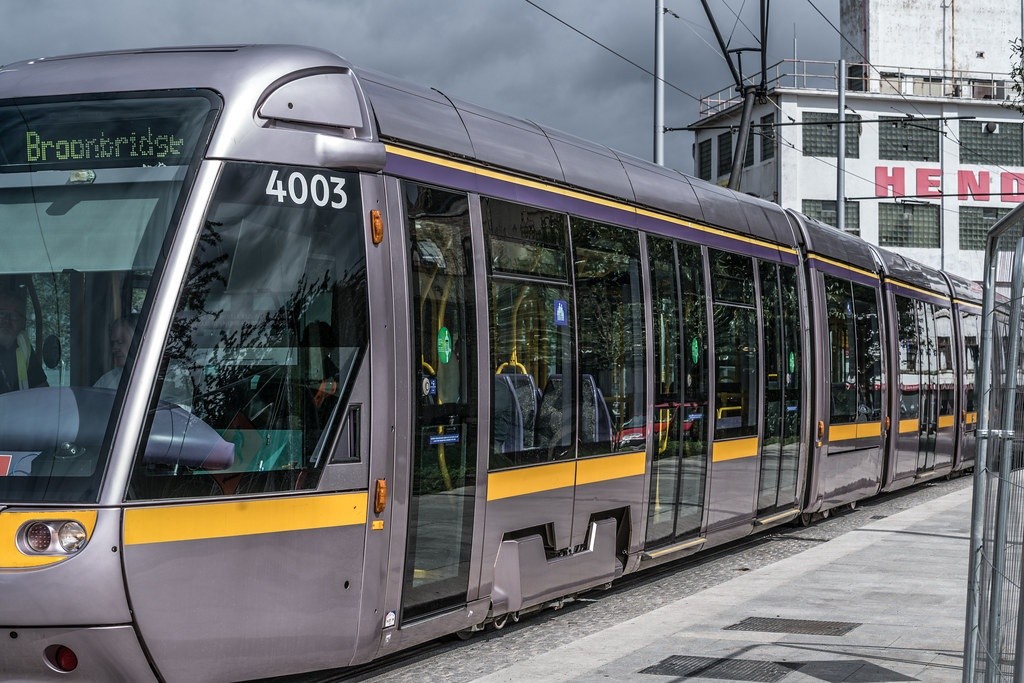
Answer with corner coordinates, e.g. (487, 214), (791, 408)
(1, 39), (1024, 683)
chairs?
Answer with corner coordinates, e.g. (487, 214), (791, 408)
(494, 374), (524, 454)
(507, 373), (538, 449)
(533, 373), (598, 447)
(595, 387), (613, 453)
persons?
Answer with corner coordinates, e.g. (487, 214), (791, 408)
(281, 321), (338, 429)
(684, 373), (697, 459)
(0, 287), (50, 394)
(844, 382), (861, 424)
(785, 372), (795, 387)
(92, 313), (194, 413)
(512, 379), (562, 449)
